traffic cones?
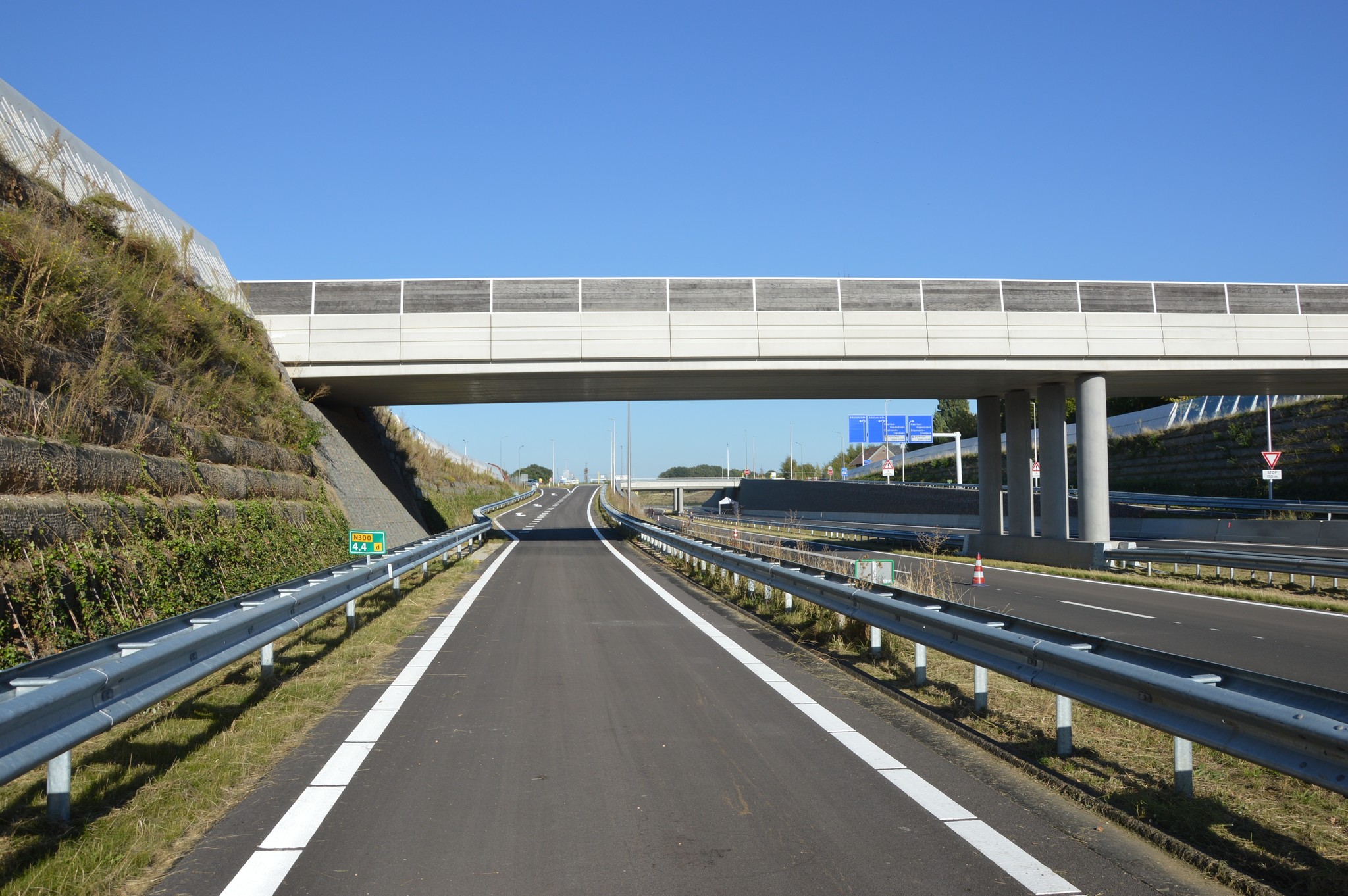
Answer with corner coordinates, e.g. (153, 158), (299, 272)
(657, 515), (660, 522)
(733, 526), (738, 538)
(681, 519), (686, 529)
(966, 552), (990, 587)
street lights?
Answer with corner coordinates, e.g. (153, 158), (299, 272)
(726, 443), (729, 477)
(607, 418), (615, 497)
(519, 445), (525, 476)
(550, 439), (554, 487)
(744, 429), (748, 478)
(463, 440), (467, 456)
(790, 423), (793, 480)
(833, 431), (846, 481)
(500, 435), (509, 468)
(885, 400), (893, 484)
(796, 442), (803, 481)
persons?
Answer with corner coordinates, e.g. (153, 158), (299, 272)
(647, 507), (654, 520)
(688, 511), (694, 522)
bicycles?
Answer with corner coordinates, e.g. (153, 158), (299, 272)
(647, 514), (654, 519)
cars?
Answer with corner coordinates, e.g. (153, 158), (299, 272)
(523, 479), (544, 488)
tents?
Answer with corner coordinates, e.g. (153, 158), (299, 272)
(719, 496), (736, 515)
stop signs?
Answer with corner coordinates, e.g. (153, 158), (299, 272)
(828, 470), (833, 475)
(744, 470), (750, 475)
